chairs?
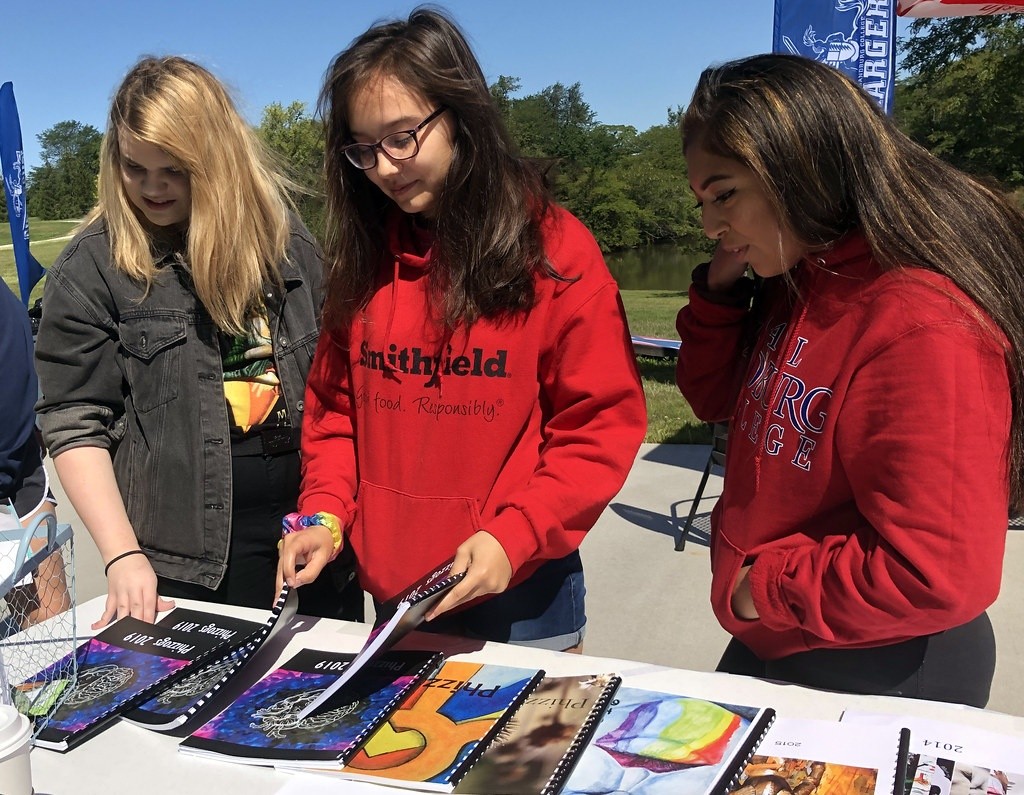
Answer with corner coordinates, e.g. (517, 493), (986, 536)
(675, 422), (729, 552)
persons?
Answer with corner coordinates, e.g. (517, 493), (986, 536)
(2, 280), (72, 638)
(676, 55), (1024, 708)
(272, 3), (648, 652)
(31, 57), (366, 630)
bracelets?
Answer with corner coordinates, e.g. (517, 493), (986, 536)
(277, 511), (344, 563)
(105, 550), (149, 577)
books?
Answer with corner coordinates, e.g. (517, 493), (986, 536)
(179, 645), (444, 772)
(558, 687), (1023, 795)
(112, 579), (298, 733)
(272, 653), (546, 790)
(8, 615), (225, 759)
(449, 677), (621, 795)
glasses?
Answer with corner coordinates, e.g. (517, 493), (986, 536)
(339, 104), (447, 170)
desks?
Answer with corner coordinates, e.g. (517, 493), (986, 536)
(0, 593), (1024, 795)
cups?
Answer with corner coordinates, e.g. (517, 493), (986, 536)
(0, 704), (36, 795)
